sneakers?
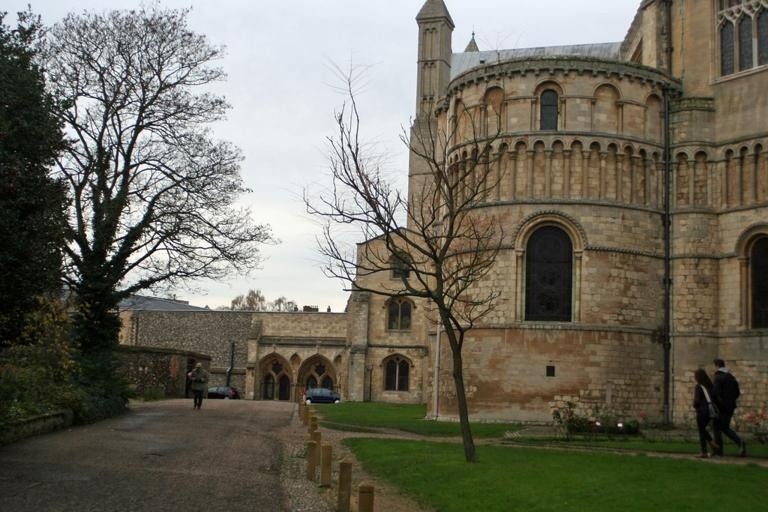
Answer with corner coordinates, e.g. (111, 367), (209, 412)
(693, 440), (724, 458)
(739, 440), (747, 457)
(193, 403), (200, 409)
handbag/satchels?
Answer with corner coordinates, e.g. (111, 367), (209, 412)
(708, 402), (720, 419)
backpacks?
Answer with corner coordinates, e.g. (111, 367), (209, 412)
(717, 372), (741, 400)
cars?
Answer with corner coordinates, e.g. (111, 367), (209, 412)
(303, 387), (341, 404)
(208, 386), (241, 399)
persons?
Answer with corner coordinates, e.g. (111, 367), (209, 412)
(187, 362), (211, 410)
(692, 368), (724, 459)
(710, 358), (747, 458)
